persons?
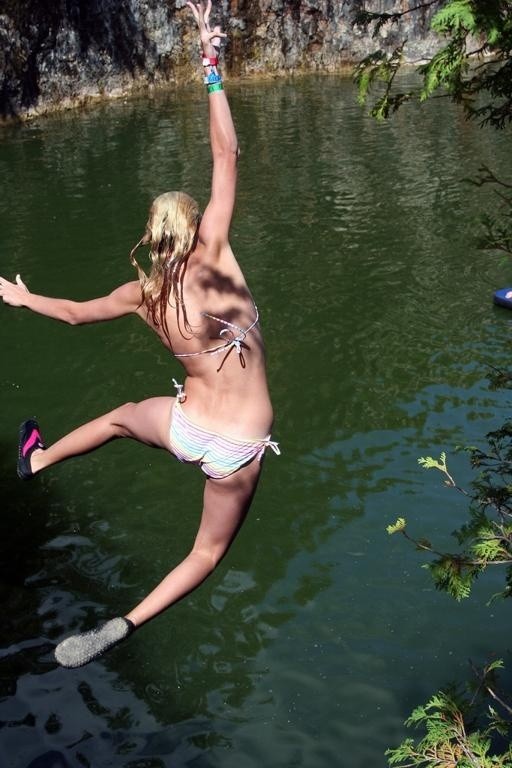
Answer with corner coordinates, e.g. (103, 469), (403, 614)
(0, 0), (259, 670)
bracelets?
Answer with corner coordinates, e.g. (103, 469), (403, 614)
(201, 51), (225, 96)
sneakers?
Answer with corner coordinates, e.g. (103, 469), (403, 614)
(16, 418), (47, 482)
(53, 616), (134, 670)
